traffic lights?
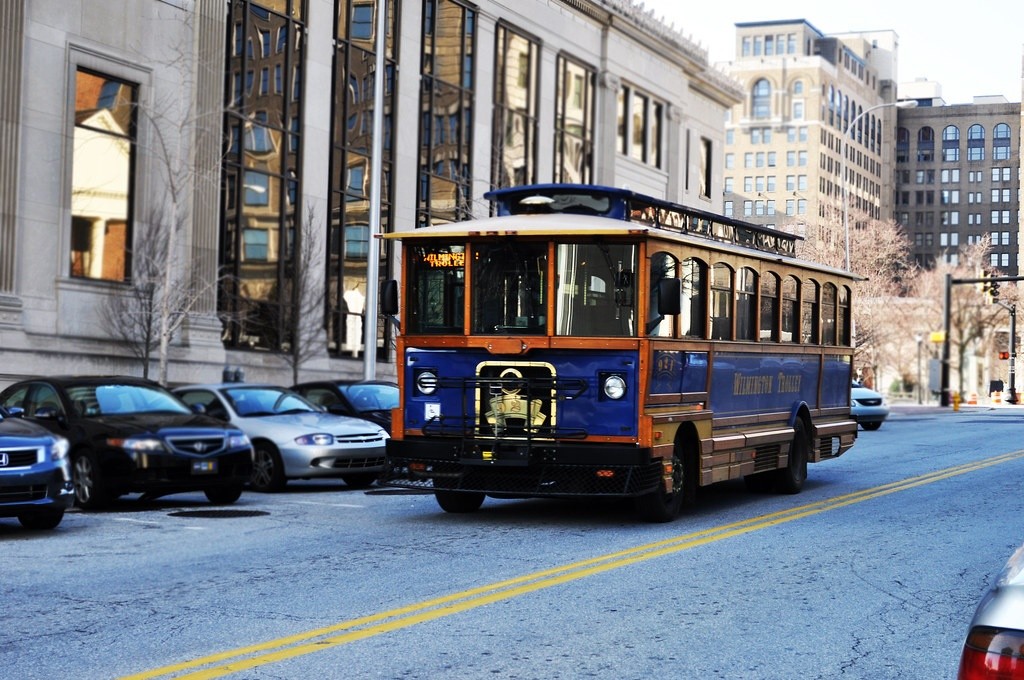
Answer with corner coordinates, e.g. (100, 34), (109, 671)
(999, 351), (1008, 360)
(989, 296), (999, 305)
(980, 270), (990, 293)
(991, 274), (1000, 299)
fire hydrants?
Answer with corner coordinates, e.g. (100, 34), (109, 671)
(950, 393), (962, 411)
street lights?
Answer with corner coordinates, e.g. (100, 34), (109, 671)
(841, 99), (921, 271)
(915, 334), (922, 404)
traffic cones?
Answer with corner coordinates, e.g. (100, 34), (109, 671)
(970, 394), (977, 405)
(994, 391), (1001, 404)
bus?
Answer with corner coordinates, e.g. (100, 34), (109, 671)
(374, 181), (858, 516)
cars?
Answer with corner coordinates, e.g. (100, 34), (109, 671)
(0, 400), (77, 531)
(954, 541), (1024, 680)
(849, 377), (889, 432)
(0, 373), (256, 512)
(284, 378), (426, 480)
(173, 383), (393, 493)
